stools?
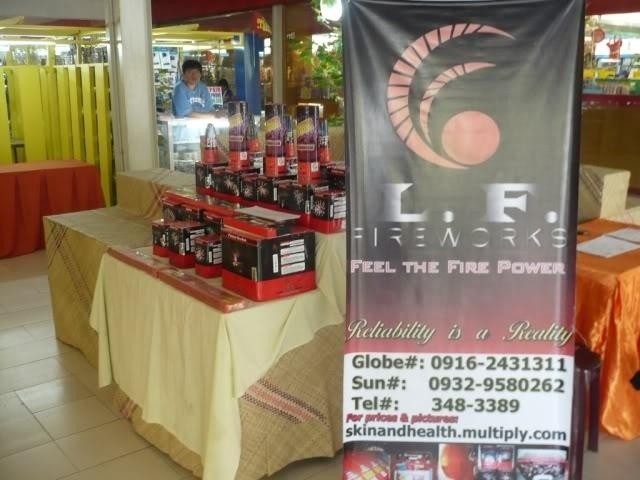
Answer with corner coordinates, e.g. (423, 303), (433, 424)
(576, 347), (601, 480)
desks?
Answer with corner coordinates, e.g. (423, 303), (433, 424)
(575, 218), (640, 441)
(0, 159), (97, 257)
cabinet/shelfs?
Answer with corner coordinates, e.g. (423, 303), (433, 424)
(43, 168), (346, 480)
(159, 116), (260, 171)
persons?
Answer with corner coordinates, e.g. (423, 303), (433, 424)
(218, 78), (234, 104)
(172, 59), (227, 120)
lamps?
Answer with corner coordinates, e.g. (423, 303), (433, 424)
(320, 0), (343, 22)
